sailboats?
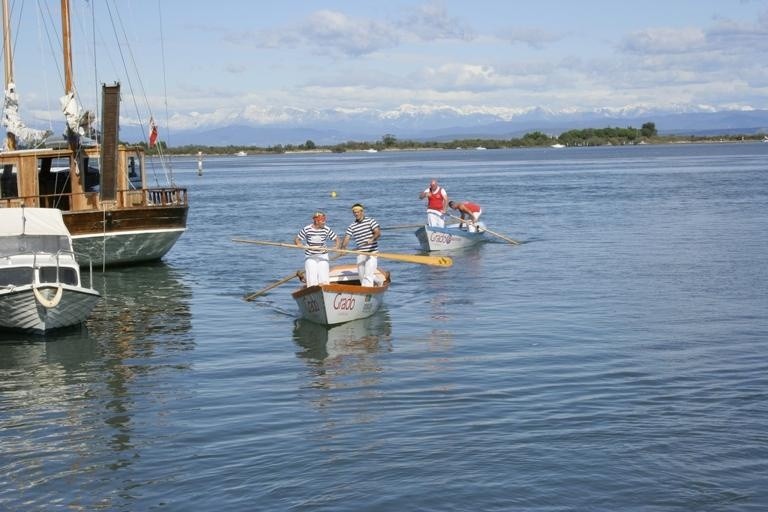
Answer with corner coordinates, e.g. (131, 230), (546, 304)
(0, 1), (191, 267)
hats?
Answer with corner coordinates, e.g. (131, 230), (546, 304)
(312, 211), (326, 221)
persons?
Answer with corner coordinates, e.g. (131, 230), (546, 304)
(419, 179), (449, 230)
(340, 203), (382, 288)
(294, 211), (340, 287)
(448, 201), (483, 233)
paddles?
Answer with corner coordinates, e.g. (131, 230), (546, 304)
(231, 239), (453, 267)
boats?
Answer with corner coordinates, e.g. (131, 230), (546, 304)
(0, 205), (101, 334)
(291, 262), (392, 325)
(288, 314), (391, 357)
(414, 221), (487, 252)
